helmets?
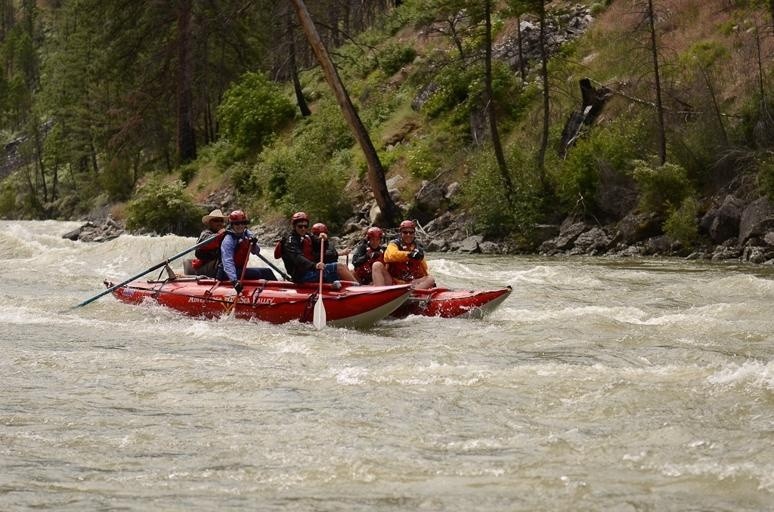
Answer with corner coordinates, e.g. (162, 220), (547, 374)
(292, 212), (309, 229)
(399, 220), (415, 231)
(229, 210), (246, 222)
(311, 223), (328, 236)
(366, 227), (383, 244)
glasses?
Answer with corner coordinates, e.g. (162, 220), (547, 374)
(402, 231), (415, 234)
(297, 224), (308, 229)
(234, 221), (248, 225)
(211, 218), (224, 223)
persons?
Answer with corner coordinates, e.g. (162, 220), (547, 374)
(281, 212), (357, 284)
(311, 223), (352, 264)
(372, 220), (435, 290)
(352, 226), (387, 285)
(192, 209), (231, 275)
(215, 209), (278, 295)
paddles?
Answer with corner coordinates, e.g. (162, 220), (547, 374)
(230, 242), (253, 315)
(313, 239), (325, 329)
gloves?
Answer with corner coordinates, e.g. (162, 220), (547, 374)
(368, 253), (380, 259)
(408, 251), (423, 260)
(249, 237), (258, 246)
(414, 248), (424, 261)
(232, 280), (243, 294)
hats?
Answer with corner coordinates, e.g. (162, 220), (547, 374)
(202, 209), (229, 226)
